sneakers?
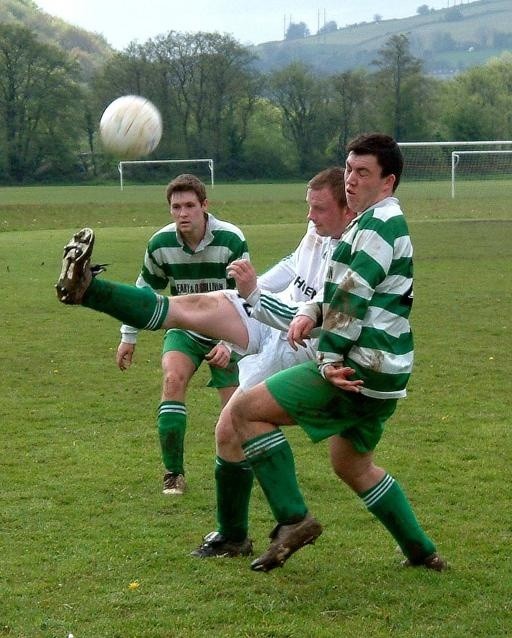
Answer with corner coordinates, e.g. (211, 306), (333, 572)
(398, 551), (445, 573)
(187, 529), (256, 560)
(54, 224), (109, 308)
(249, 510), (327, 574)
(161, 470), (189, 496)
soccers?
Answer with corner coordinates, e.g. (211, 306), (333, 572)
(98, 96), (162, 159)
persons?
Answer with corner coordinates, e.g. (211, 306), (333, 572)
(230, 132), (451, 574)
(51, 167), (360, 558)
(114, 172), (255, 497)
